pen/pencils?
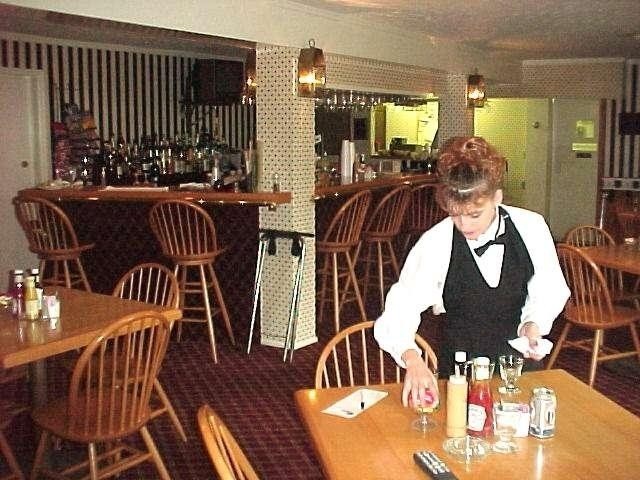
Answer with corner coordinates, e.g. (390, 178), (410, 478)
(361, 392), (364, 410)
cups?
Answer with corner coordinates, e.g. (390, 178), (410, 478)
(340, 140), (356, 184)
(40, 291), (60, 319)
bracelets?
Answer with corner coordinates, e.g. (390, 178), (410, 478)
(516, 317), (540, 337)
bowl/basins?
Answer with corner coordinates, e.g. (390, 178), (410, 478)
(495, 400), (530, 438)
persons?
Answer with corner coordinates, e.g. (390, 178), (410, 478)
(372, 133), (571, 416)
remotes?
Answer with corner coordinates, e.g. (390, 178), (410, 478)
(413, 450), (460, 480)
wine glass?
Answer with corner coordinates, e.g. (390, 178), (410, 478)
(496, 354), (524, 394)
(407, 390), (440, 432)
(492, 411), (521, 455)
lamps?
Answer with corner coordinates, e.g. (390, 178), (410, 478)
(468, 67), (487, 109)
(297, 38), (327, 100)
(245, 49), (256, 97)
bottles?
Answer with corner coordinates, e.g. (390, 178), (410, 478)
(317, 151), (340, 187)
(455, 351), (466, 377)
(530, 385), (557, 438)
(31, 269), (44, 316)
(359, 155), (377, 178)
(25, 276), (39, 320)
(444, 368), (467, 435)
(77, 135), (227, 178)
(467, 356), (493, 438)
(11, 269), (25, 319)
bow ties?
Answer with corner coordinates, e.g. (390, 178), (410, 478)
(474, 233), (510, 257)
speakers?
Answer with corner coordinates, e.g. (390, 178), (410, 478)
(618, 112), (640, 135)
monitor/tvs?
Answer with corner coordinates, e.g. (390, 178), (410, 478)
(191, 58), (244, 106)
